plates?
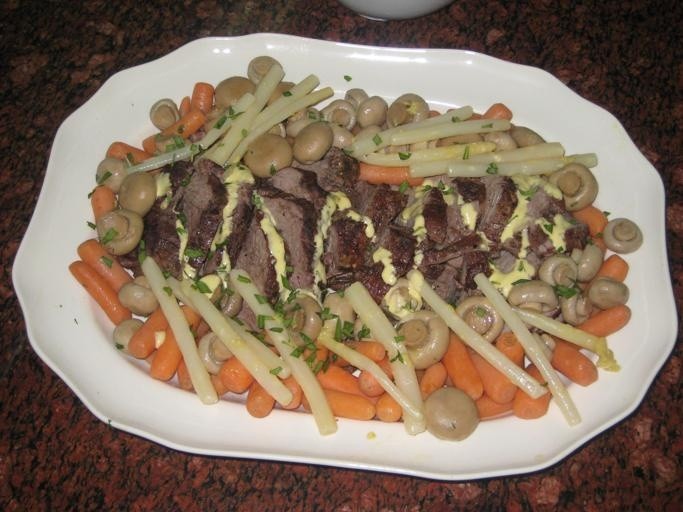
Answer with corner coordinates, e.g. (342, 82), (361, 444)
(9, 30), (680, 480)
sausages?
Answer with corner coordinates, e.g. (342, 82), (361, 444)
(69, 78), (632, 422)
